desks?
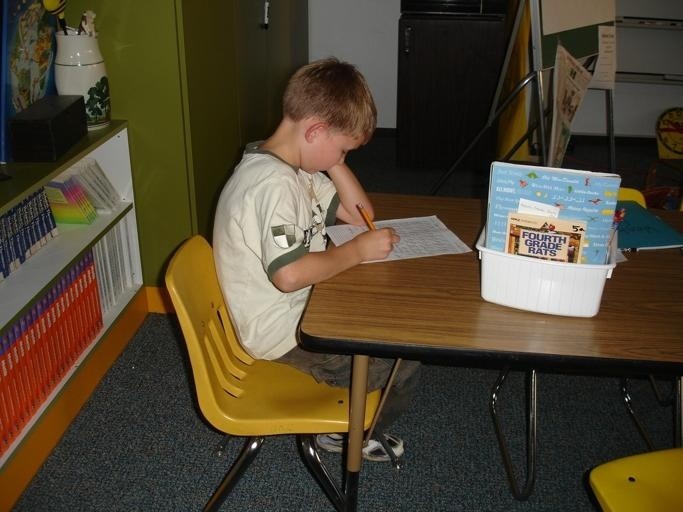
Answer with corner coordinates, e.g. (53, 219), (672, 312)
(289, 186), (682, 499)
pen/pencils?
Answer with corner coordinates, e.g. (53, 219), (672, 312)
(355, 203), (376, 231)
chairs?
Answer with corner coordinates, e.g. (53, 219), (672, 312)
(166, 236), (381, 512)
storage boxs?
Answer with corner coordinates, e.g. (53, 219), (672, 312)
(469, 218), (618, 320)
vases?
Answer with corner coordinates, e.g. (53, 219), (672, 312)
(53, 27), (114, 131)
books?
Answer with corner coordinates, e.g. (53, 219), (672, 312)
(505, 211), (587, 263)
(42, 157), (120, 225)
(1, 219), (134, 455)
(1, 185), (60, 281)
(613, 200), (683, 253)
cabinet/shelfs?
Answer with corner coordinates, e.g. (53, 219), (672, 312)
(392, 15), (519, 182)
(71, 0), (311, 315)
(0, 121), (149, 510)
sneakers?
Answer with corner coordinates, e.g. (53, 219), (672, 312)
(315, 428), (405, 464)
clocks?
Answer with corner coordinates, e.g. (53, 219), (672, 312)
(656, 107), (683, 160)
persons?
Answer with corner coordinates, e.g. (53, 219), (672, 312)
(212, 56), (421, 462)
